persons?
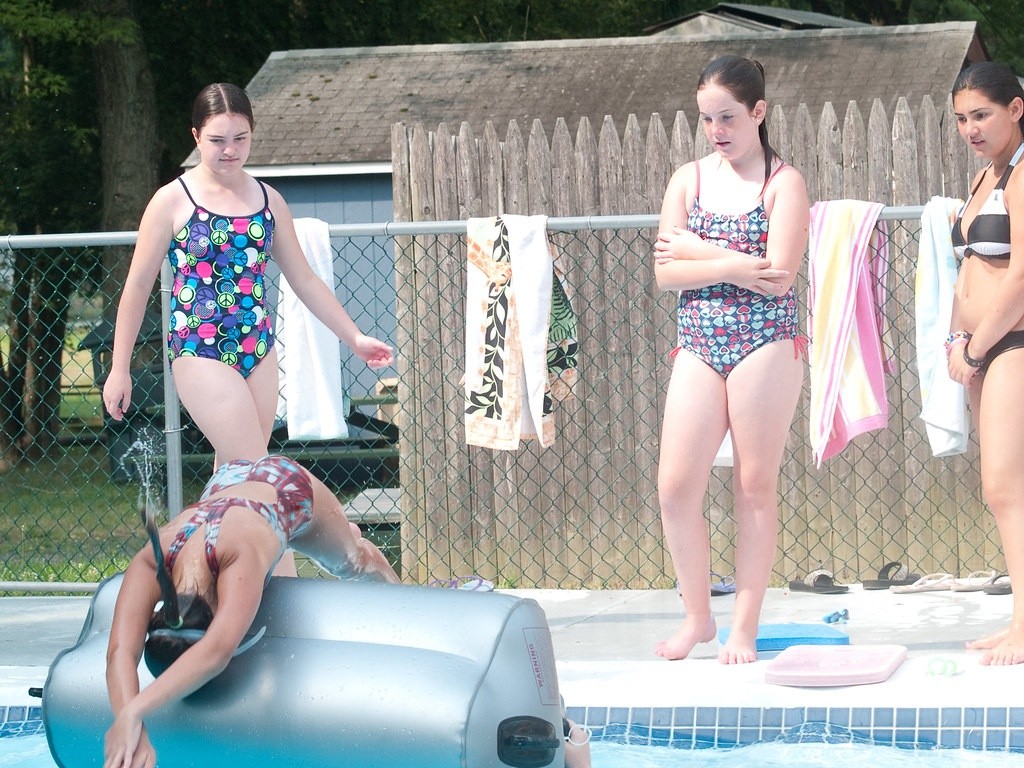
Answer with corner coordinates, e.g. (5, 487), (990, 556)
(101, 82), (396, 579)
(650, 53), (810, 667)
(562, 718), (592, 768)
(100, 452), (404, 768)
(947, 58), (1024, 668)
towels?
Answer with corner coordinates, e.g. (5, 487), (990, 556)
(912, 193), (975, 464)
(802, 196), (898, 473)
(268, 216), (353, 445)
(461, 210), (581, 455)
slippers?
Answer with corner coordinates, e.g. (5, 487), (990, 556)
(888, 572), (952, 592)
(983, 574), (1012, 595)
(711, 573), (736, 595)
(789, 569), (849, 594)
(863, 562), (919, 589)
(429, 576), (494, 593)
(953, 570), (998, 591)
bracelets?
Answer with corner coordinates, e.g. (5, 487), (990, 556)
(943, 330), (973, 350)
(945, 337), (970, 358)
(962, 341), (987, 367)
